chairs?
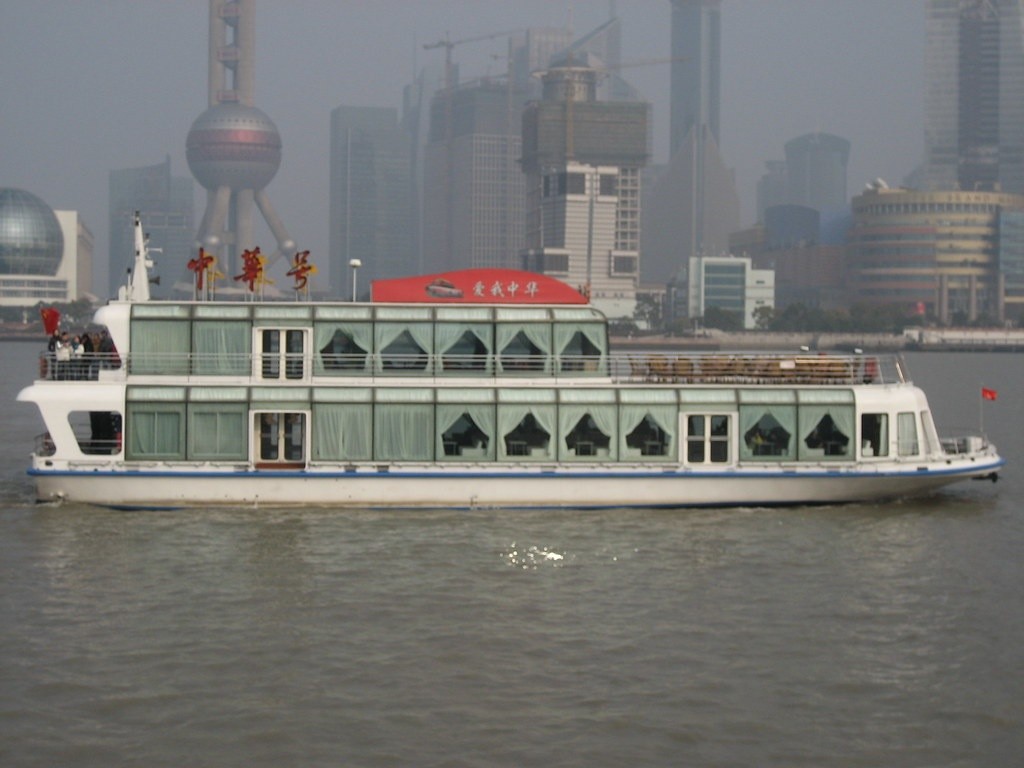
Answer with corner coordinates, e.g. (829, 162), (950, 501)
(626, 353), (867, 385)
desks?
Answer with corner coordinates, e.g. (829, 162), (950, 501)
(444, 441), (457, 456)
(510, 440), (527, 455)
(758, 442), (775, 455)
(577, 441), (594, 456)
(644, 440), (662, 456)
(824, 441), (843, 455)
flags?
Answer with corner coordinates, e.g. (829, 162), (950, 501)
(40, 308), (61, 335)
(982, 386), (998, 400)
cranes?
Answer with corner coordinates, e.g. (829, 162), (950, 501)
(420, 24), (517, 88)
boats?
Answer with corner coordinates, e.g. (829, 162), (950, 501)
(16, 209), (1006, 512)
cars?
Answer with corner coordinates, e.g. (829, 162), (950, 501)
(426, 276), (465, 299)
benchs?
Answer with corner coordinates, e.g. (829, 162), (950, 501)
(804, 442), (825, 457)
(457, 438), (481, 457)
(526, 440), (547, 456)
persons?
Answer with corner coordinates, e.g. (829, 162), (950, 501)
(48, 329), (121, 381)
